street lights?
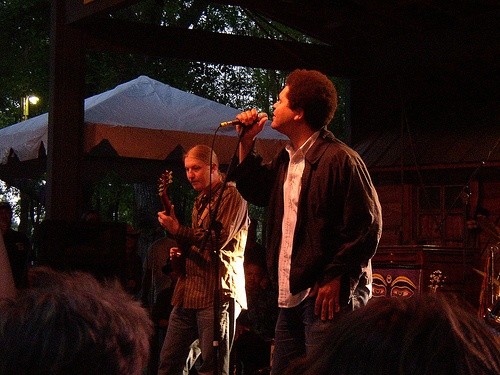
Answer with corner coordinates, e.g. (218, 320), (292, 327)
(19, 88), (40, 233)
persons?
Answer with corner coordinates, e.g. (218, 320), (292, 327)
(234, 68), (381, 375)
(156, 144), (247, 375)
(0, 202), (500, 375)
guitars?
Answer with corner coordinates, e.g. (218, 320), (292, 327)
(156, 168), (187, 287)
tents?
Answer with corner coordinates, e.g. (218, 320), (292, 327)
(0, 77), (289, 181)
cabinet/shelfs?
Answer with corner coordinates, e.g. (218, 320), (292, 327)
(41, 220), (138, 283)
(371, 244), (479, 302)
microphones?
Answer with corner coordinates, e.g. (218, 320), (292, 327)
(220, 112), (268, 127)
